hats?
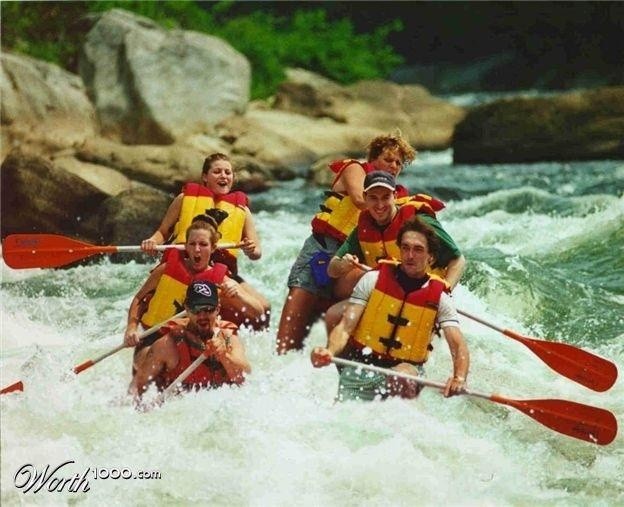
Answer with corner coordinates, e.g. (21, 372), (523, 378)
(186, 280), (218, 314)
(364, 170), (396, 193)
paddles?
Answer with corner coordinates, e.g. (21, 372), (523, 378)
(350, 260), (618, 393)
(317, 352), (616, 444)
(3, 234), (251, 270)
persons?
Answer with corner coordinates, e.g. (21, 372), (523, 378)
(140, 151), (271, 331)
(324, 169), (467, 336)
(275, 132), (421, 357)
(124, 280), (251, 412)
(306, 220), (471, 401)
(123, 221), (264, 347)
